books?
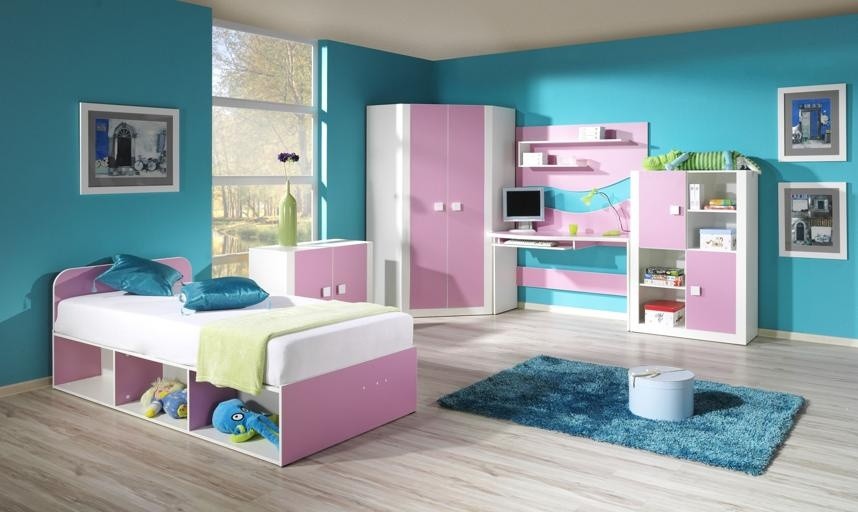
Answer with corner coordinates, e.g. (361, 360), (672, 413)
(703, 199), (739, 210)
(688, 183), (701, 210)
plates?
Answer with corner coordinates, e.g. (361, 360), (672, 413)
(602, 230), (621, 236)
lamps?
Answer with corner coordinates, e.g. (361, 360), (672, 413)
(582, 188), (624, 236)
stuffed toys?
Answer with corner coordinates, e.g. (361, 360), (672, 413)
(213, 398), (280, 449)
(645, 149), (764, 174)
(140, 375), (188, 419)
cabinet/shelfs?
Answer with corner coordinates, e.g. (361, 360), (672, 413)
(248, 238), (374, 303)
(365, 104), (517, 319)
(629, 170), (759, 346)
(491, 123), (649, 315)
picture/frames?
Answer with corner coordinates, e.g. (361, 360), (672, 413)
(777, 182), (847, 260)
(79, 102), (180, 195)
(778, 83), (847, 163)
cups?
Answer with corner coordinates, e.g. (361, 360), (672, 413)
(569, 224), (577, 237)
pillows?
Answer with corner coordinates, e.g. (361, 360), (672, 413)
(178, 277), (270, 311)
(94, 254), (183, 297)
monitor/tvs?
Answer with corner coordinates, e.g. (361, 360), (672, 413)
(502, 186), (545, 233)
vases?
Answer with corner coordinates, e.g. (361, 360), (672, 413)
(278, 179), (297, 247)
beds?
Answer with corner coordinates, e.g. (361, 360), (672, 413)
(52, 257), (419, 468)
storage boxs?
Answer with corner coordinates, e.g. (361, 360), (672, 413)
(643, 301), (685, 329)
(699, 228), (736, 251)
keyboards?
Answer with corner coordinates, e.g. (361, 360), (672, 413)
(505, 240), (560, 248)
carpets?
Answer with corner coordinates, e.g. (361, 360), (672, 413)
(437, 354), (806, 477)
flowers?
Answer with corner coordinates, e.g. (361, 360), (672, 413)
(277, 152), (300, 180)
